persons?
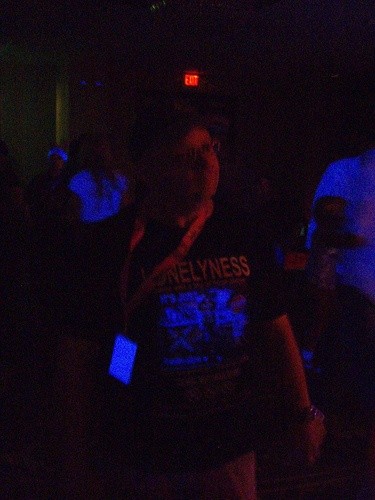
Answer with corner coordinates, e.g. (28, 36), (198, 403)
(2, 108), (130, 251)
(69, 124), (329, 499)
(309, 134), (374, 342)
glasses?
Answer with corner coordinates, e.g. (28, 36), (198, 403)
(158, 136), (222, 173)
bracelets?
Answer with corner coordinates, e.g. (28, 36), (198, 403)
(292, 404), (323, 427)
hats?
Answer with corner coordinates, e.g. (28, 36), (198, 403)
(129, 96), (232, 162)
